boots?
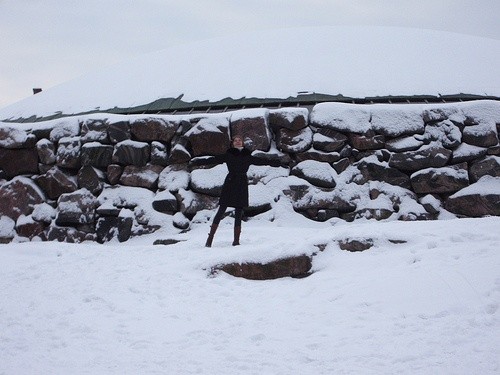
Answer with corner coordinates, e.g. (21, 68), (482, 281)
(232, 226), (241, 246)
(206, 223), (218, 247)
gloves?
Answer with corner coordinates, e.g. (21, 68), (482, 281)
(280, 161), (292, 168)
(187, 161), (199, 167)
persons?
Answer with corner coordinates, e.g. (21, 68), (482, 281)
(188, 135), (290, 247)
